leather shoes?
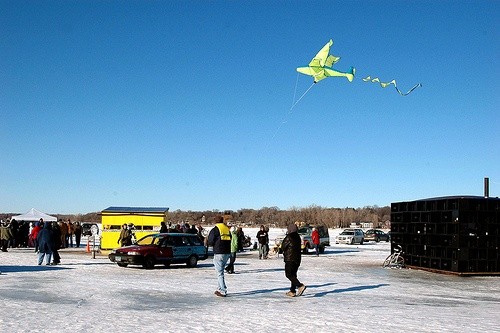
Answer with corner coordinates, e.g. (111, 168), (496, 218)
(230, 270), (236, 273)
(225, 267), (231, 273)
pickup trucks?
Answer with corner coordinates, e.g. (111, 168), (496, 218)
(275, 222), (330, 254)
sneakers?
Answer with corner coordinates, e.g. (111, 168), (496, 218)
(215, 290), (220, 294)
(217, 291), (226, 296)
(3, 249), (8, 251)
(297, 283), (306, 294)
(286, 290), (296, 296)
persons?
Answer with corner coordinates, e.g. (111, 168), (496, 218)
(37, 221), (54, 265)
(263, 224), (269, 259)
(58, 218), (83, 248)
(311, 227), (321, 255)
(207, 215), (232, 297)
(224, 227), (239, 274)
(256, 224), (269, 260)
(281, 223), (306, 297)
(117, 223), (132, 247)
(0, 219), (43, 252)
(159, 221), (203, 235)
(51, 222), (61, 266)
(236, 227), (245, 252)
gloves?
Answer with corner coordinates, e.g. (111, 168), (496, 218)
(122, 237), (125, 241)
(117, 239), (120, 244)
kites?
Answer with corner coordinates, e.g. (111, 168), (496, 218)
(296, 39), (423, 97)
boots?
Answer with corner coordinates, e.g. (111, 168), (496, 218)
(51, 259), (57, 264)
(56, 257), (60, 262)
(263, 255), (267, 258)
(259, 255), (261, 259)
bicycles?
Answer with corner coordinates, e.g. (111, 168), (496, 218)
(382, 244), (405, 270)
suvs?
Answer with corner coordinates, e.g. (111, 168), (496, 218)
(335, 230), (364, 244)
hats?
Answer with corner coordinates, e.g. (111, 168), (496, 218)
(35, 221), (40, 225)
(287, 223), (298, 231)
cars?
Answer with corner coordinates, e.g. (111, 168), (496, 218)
(365, 229), (389, 242)
(81, 222), (99, 236)
(108, 232), (208, 268)
